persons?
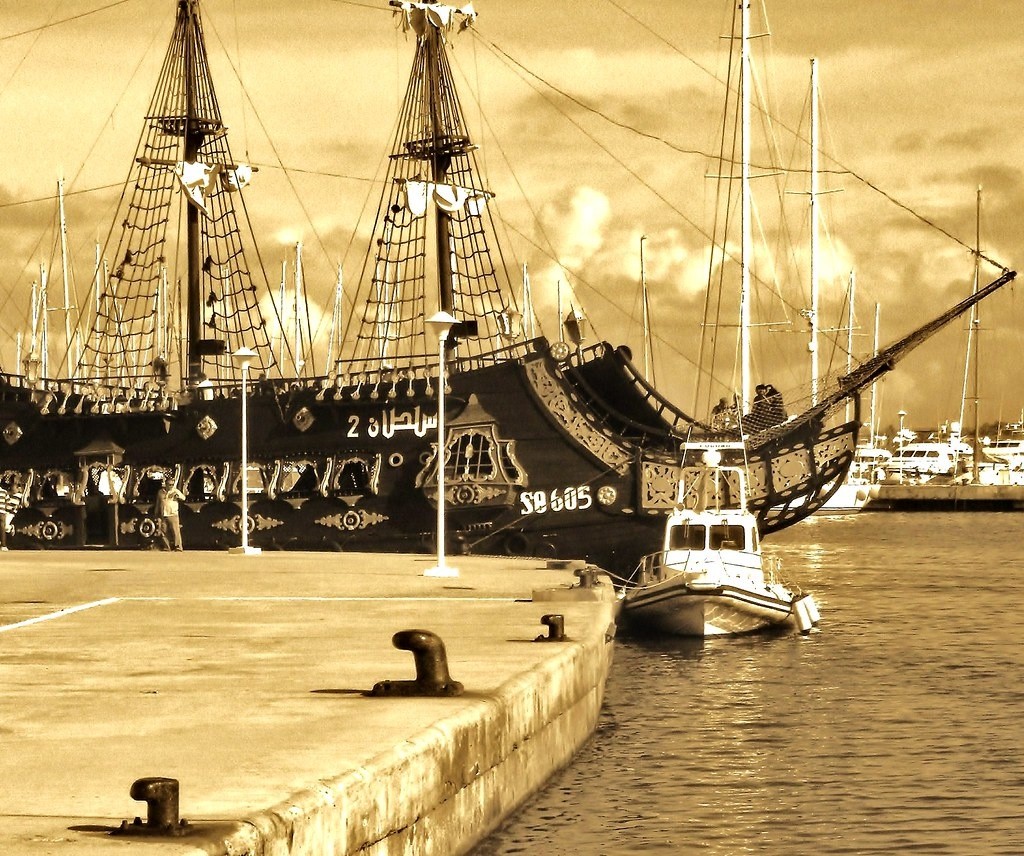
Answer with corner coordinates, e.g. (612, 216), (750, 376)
(153, 478), (186, 551)
(712, 384), (787, 440)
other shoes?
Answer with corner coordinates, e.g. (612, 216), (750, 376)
(10, 524), (15, 536)
(164, 548), (170, 550)
(1, 546), (9, 551)
(175, 548), (182, 552)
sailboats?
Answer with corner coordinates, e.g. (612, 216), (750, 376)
(0, 0), (1019, 567)
(622, 1), (820, 642)
(634, 1), (1024, 513)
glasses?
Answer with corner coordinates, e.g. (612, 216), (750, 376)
(168, 484), (174, 486)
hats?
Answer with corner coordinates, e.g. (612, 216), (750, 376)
(13, 493), (22, 498)
